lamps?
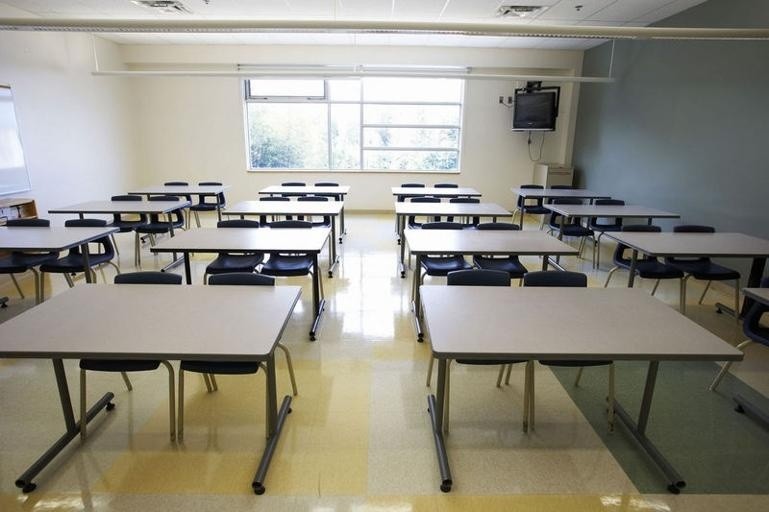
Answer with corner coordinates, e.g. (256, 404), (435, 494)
(80, 21), (357, 81)
(358, 29), (622, 82)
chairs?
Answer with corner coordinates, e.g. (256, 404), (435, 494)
(161, 181), (225, 228)
(472, 222), (529, 286)
(649, 226), (742, 323)
(506, 268), (617, 437)
(77, 269), (213, 443)
(259, 219), (326, 319)
(393, 183), (480, 268)
(134, 195), (195, 265)
(604, 223), (683, 315)
(410, 221), (473, 302)
(424, 267), (535, 438)
(259, 181), (343, 267)
(38, 218), (122, 303)
(509, 183), (581, 227)
(579, 198), (627, 269)
(706, 274), (769, 394)
(538, 198), (596, 269)
(98, 195), (155, 266)
(202, 220), (266, 285)
(177, 270), (298, 444)
(1, 218), (71, 300)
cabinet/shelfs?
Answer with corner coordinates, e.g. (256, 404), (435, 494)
(532, 160), (576, 222)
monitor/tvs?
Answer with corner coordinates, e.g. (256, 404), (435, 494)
(513, 92), (556, 131)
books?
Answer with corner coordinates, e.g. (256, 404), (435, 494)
(0, 205), (24, 226)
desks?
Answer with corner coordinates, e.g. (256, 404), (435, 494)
(220, 200), (344, 279)
(402, 228), (581, 344)
(127, 183), (230, 221)
(602, 231), (769, 320)
(256, 184), (349, 244)
(47, 199), (193, 276)
(542, 203), (682, 272)
(393, 202), (513, 279)
(742, 284), (769, 311)
(2, 225), (121, 304)
(1, 280), (303, 495)
(149, 227), (333, 338)
(416, 283), (747, 494)
(2, 196), (37, 218)
(391, 186), (483, 246)
(510, 187), (613, 231)
